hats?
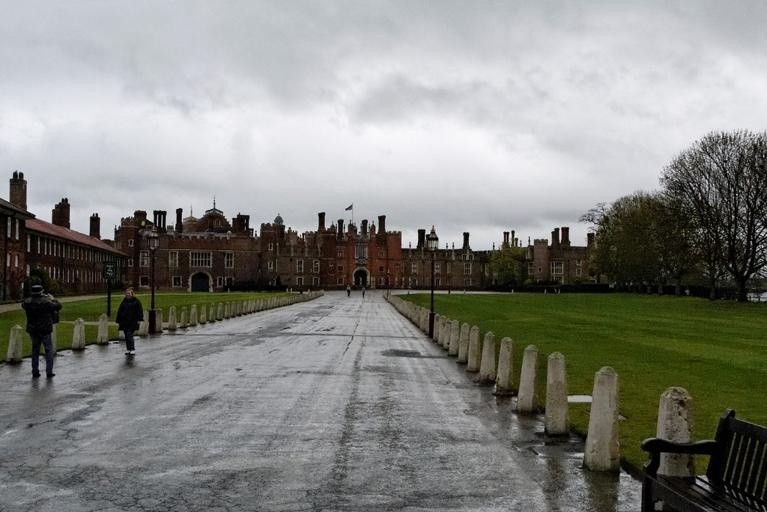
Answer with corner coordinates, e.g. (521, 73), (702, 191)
(31, 285), (44, 295)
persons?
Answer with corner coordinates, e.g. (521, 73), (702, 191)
(21, 284), (63, 377)
(346, 284), (351, 296)
(361, 285), (365, 298)
(115, 288), (144, 357)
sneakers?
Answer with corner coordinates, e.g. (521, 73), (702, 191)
(34, 373), (41, 378)
(125, 350), (135, 354)
(47, 373), (55, 378)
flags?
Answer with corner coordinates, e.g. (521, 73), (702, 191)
(344, 204), (352, 211)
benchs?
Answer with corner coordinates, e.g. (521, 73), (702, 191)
(640, 409), (767, 512)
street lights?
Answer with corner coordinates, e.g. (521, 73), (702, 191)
(426, 225), (439, 339)
(148, 224), (160, 309)
